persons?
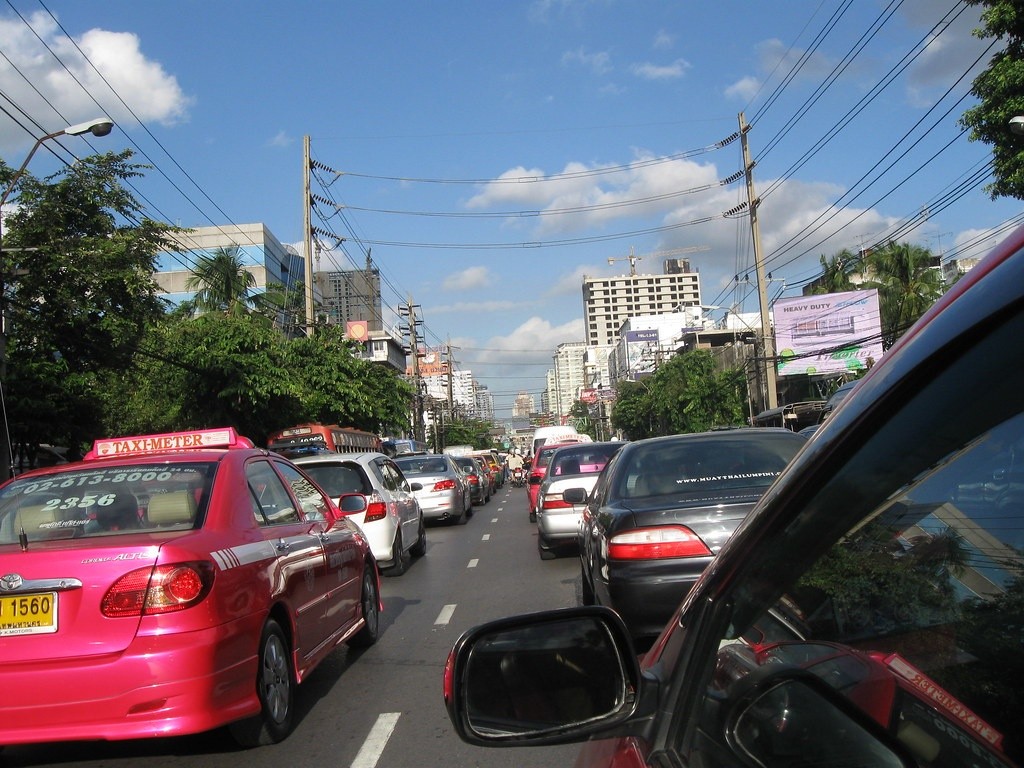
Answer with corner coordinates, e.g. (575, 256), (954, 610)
(505, 447), (533, 481)
(96, 493), (137, 531)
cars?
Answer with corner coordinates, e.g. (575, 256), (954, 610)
(562, 427), (812, 655)
(440, 222), (1024, 767)
(0, 426), (387, 749)
(382, 453), (473, 525)
(526, 440), (645, 561)
(739, 624), (1017, 766)
(254, 452), (427, 578)
(420, 453), (491, 506)
(461, 449), (510, 497)
(522, 443), (610, 524)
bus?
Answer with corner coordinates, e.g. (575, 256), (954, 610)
(752, 378), (862, 435)
(382, 437), (429, 462)
(267, 421), (386, 464)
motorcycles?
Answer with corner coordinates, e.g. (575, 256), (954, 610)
(509, 466), (526, 488)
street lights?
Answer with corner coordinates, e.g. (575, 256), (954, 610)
(700, 305), (778, 410)
(1, 115), (114, 202)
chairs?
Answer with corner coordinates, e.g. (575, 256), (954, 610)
(645, 466), (683, 495)
(13, 504), (65, 539)
(148, 490), (196, 529)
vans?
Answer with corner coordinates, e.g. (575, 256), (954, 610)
(531, 425), (593, 457)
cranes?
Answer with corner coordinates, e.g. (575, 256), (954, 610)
(608, 244), (712, 279)
